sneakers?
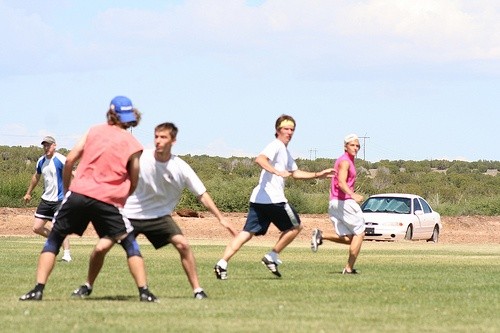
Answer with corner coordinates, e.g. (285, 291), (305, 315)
(262, 251), (283, 277)
(19, 285), (43, 301)
(193, 287), (207, 298)
(311, 229), (323, 253)
(72, 282), (92, 298)
(138, 286), (156, 301)
(343, 268), (357, 275)
(213, 258), (228, 280)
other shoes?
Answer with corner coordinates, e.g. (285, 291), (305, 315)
(62, 250), (72, 262)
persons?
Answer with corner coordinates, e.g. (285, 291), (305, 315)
(311, 136), (366, 272)
(24, 136), (71, 261)
(216, 115), (337, 279)
(20, 96), (157, 302)
(73, 123), (238, 298)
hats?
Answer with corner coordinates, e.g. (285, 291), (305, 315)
(41, 136), (55, 145)
(110, 95), (137, 123)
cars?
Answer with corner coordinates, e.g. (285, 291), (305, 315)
(360, 193), (442, 242)
